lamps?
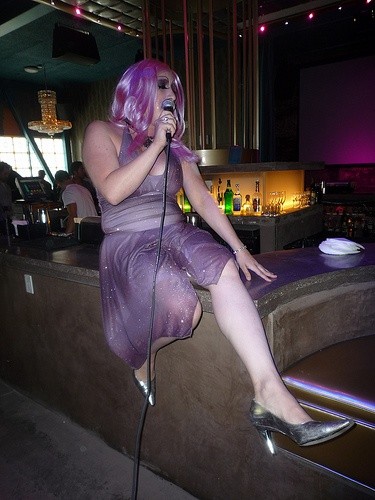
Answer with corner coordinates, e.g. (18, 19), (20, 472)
(28, 66), (73, 139)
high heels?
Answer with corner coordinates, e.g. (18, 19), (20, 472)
(132, 369), (156, 407)
(248, 399), (354, 456)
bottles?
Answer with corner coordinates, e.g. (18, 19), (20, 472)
(233, 183), (242, 216)
(252, 180), (261, 215)
(223, 180), (234, 215)
(241, 195), (252, 216)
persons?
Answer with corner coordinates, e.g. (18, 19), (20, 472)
(82, 57), (356, 457)
(0, 159), (102, 256)
(232, 245), (247, 254)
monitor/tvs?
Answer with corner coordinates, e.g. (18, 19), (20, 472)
(15, 177), (47, 201)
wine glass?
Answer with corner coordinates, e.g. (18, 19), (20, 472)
(291, 191), (310, 208)
(268, 192), (286, 217)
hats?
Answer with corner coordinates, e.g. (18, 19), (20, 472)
(39, 170), (46, 175)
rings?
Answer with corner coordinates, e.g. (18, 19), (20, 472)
(164, 117), (169, 124)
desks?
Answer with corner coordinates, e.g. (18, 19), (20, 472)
(185, 162), (323, 254)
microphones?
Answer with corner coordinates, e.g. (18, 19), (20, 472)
(162, 99), (175, 141)
